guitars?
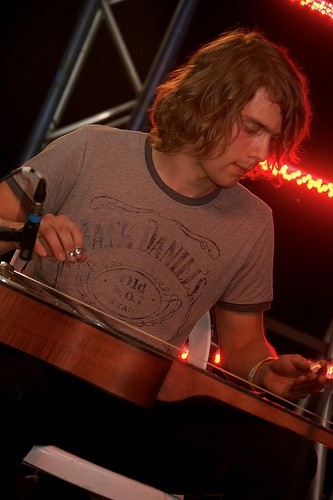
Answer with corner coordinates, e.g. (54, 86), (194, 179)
(1, 254), (333, 448)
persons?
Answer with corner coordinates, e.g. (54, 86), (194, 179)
(0, 29), (333, 500)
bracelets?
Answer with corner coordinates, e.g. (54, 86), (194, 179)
(246, 353), (277, 389)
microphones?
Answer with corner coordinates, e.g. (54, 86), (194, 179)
(19, 178), (46, 260)
